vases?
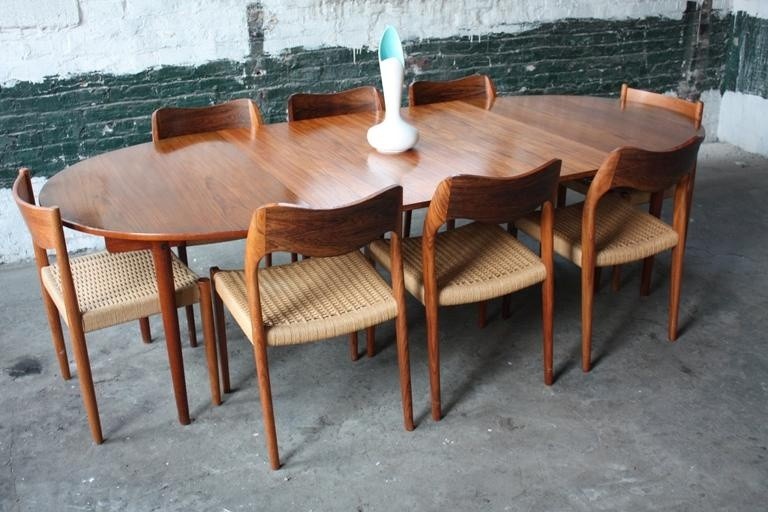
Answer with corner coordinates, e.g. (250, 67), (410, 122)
(366, 22), (420, 154)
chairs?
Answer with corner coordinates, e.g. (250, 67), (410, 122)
(363, 158), (562, 420)
(209, 185), (415, 470)
(408, 74), (495, 107)
(12, 74), (705, 471)
(152, 99), (258, 144)
(505, 137), (705, 366)
(613, 83), (705, 295)
(287, 86), (383, 120)
(11, 167), (222, 445)
